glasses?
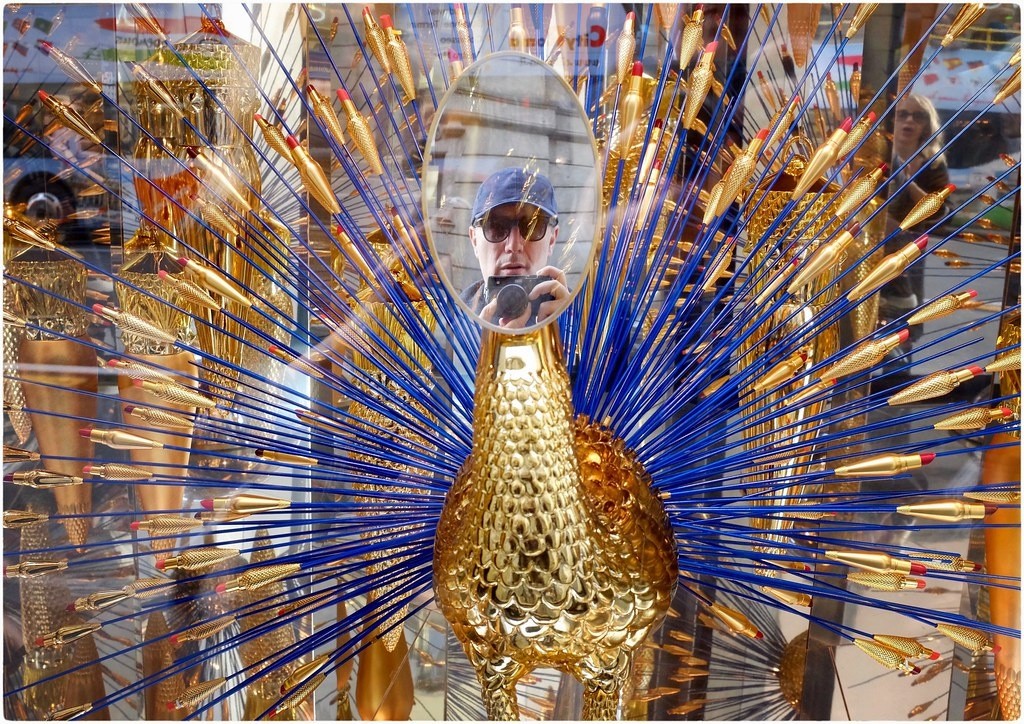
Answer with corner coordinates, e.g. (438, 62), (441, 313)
(896, 109), (928, 125)
(472, 215), (559, 243)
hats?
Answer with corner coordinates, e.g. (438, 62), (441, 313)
(470, 166), (558, 226)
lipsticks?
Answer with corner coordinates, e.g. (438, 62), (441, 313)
(3, 3), (1021, 721)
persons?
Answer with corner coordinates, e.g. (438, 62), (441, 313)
(872, 93), (955, 378)
(457, 166), (573, 331)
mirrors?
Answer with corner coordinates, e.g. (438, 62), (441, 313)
(421, 50), (605, 337)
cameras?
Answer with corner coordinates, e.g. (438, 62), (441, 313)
(485, 275), (554, 318)
